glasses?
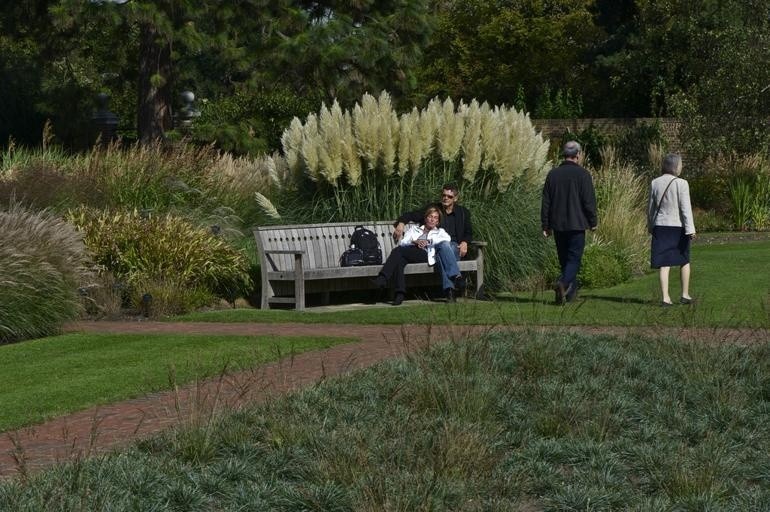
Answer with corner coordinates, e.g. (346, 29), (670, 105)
(440, 192), (460, 200)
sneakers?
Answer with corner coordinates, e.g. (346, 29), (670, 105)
(365, 275), (387, 288)
(554, 280), (567, 307)
(565, 289), (580, 302)
(661, 300), (676, 307)
(392, 292), (404, 307)
(678, 295), (695, 304)
(442, 288), (455, 303)
(457, 275), (470, 296)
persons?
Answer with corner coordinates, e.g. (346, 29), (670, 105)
(646, 153), (696, 307)
(364, 207), (451, 306)
(391, 182), (474, 301)
(540, 140), (598, 304)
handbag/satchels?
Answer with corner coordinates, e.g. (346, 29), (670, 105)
(340, 250), (366, 267)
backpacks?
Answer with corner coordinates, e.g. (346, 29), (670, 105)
(350, 224), (383, 266)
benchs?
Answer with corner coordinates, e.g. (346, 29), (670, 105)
(249, 218), (490, 311)
